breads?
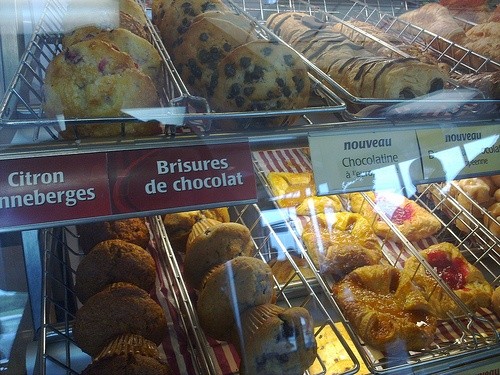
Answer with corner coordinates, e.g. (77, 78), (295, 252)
(42, 1), (500, 141)
(71, 204), (318, 375)
(267, 171), (500, 351)
